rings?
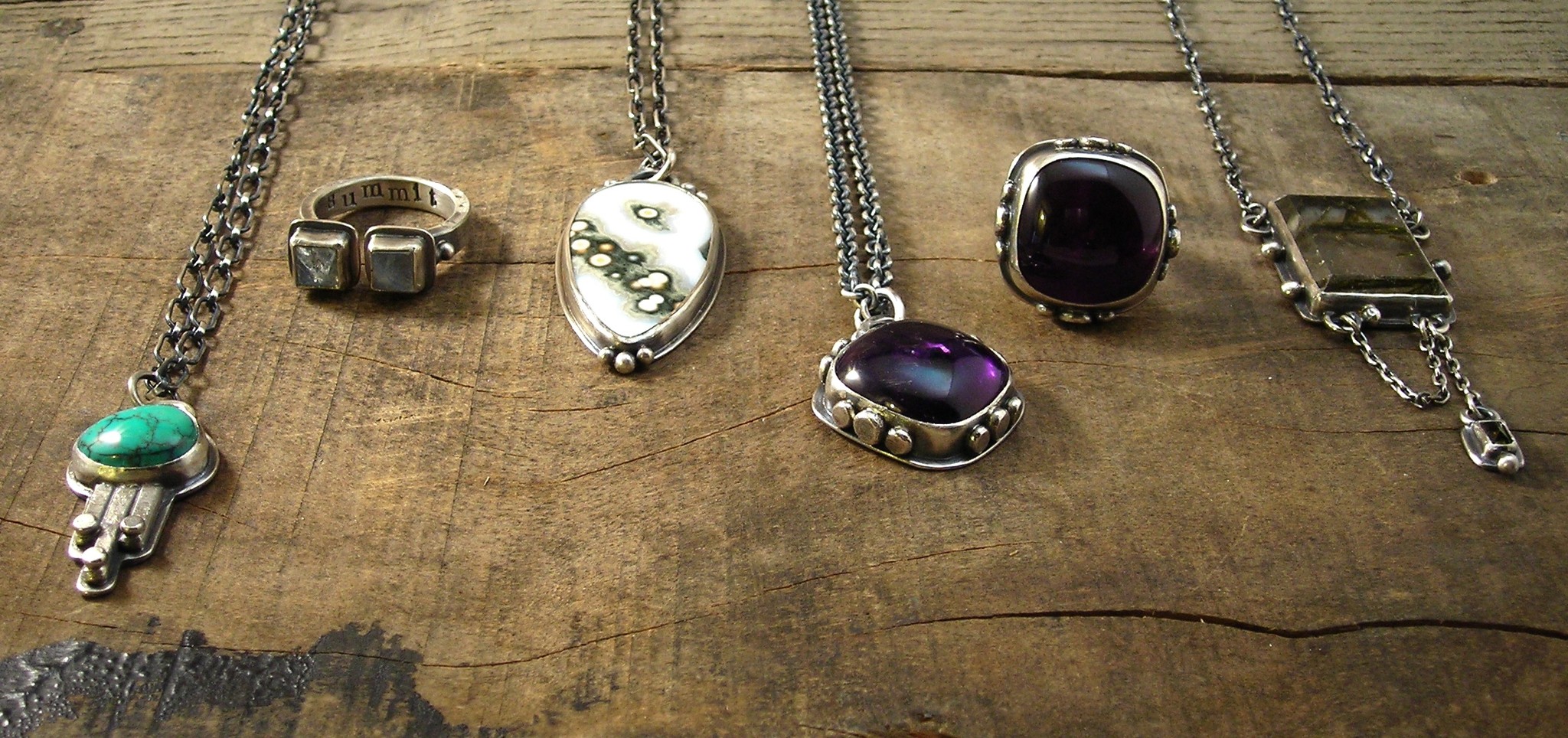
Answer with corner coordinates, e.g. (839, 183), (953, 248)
(287, 174), (472, 292)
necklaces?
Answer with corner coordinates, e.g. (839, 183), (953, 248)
(796, 0), (1027, 469)
(1161, 0), (1525, 473)
(554, 0), (726, 370)
(66, 0), (321, 595)
